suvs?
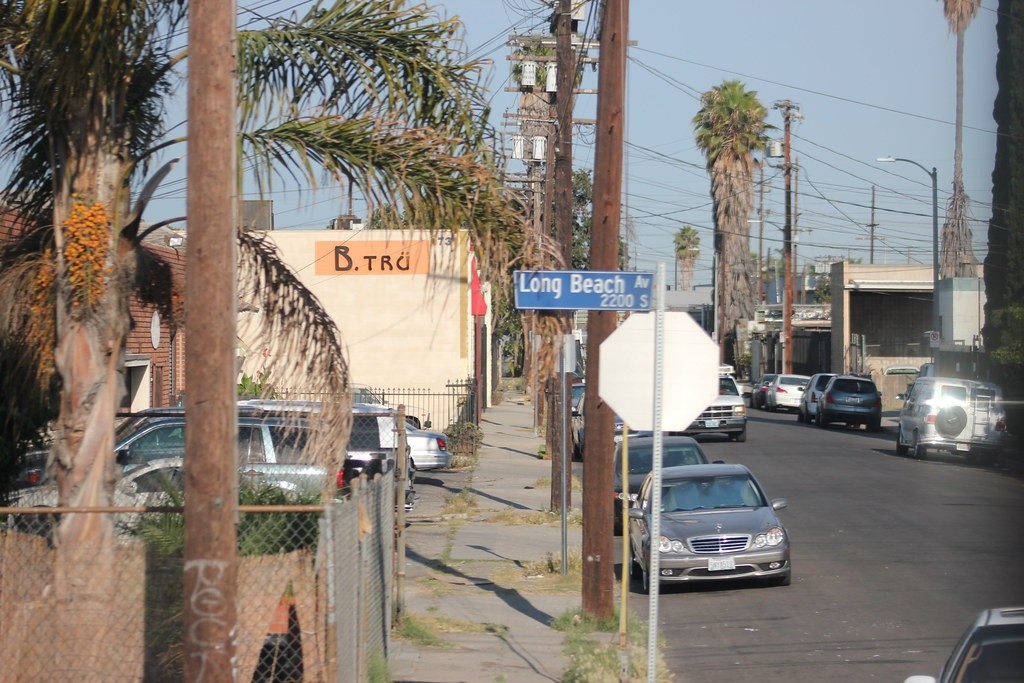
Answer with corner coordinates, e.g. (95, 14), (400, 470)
(895, 377), (1008, 467)
(815, 375), (882, 433)
(669, 373), (747, 442)
(797, 373), (839, 424)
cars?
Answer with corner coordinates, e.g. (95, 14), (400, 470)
(620, 459), (791, 593)
(614, 435), (726, 540)
(763, 374), (811, 413)
(749, 374), (777, 409)
(883, 367), (921, 400)
(570, 382), (657, 461)
(902, 605), (1024, 683)
(2, 383), (451, 552)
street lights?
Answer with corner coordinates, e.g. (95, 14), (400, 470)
(877, 158), (940, 377)
